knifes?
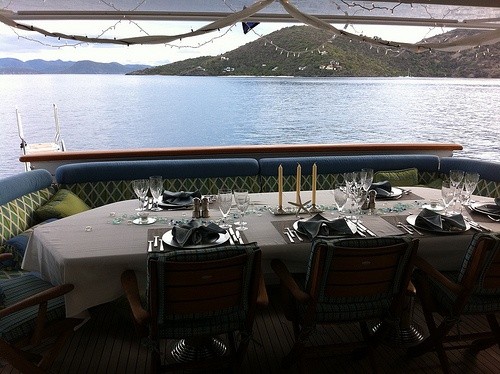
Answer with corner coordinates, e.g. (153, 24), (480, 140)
(226, 227), (244, 245)
(357, 222), (376, 237)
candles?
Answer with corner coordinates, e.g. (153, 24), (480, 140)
(312, 163), (318, 205)
(296, 162), (301, 204)
(278, 162), (283, 207)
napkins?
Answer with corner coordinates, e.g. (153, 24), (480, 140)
(298, 214), (353, 242)
(351, 181), (391, 198)
(416, 207), (466, 233)
(172, 218), (227, 247)
(475, 196), (500, 216)
(162, 189), (201, 207)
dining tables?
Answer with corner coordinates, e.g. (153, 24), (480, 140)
(19, 188), (500, 331)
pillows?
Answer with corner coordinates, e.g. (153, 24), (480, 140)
(374, 168), (418, 187)
(6, 188), (91, 261)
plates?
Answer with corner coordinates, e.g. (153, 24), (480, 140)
(158, 194), (183, 207)
(293, 218), (357, 238)
(423, 205), (444, 211)
(132, 217), (156, 225)
(471, 202), (500, 216)
(407, 214), (471, 232)
(390, 187), (402, 197)
(162, 230), (229, 248)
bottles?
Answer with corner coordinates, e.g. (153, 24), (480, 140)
(192, 197), (210, 218)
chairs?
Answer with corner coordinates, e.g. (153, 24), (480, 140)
(271, 235), (419, 374)
(121, 240), (268, 374)
(408, 232), (500, 374)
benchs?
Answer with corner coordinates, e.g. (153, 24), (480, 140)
(0, 154), (500, 374)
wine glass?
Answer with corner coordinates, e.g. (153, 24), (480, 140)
(132, 175), (163, 212)
(218, 189), (250, 232)
(443, 170), (480, 215)
(335, 168), (374, 215)
